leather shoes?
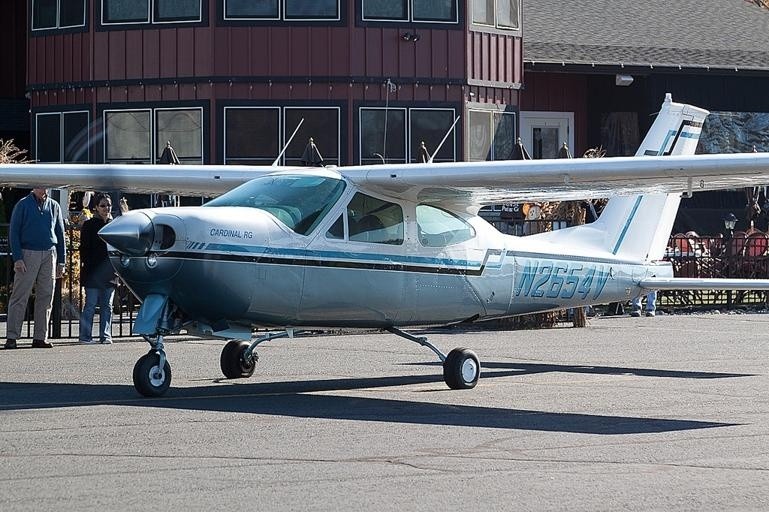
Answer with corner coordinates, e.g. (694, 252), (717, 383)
(102, 338), (111, 344)
(31, 340), (53, 348)
(79, 340), (97, 344)
(4, 339), (16, 349)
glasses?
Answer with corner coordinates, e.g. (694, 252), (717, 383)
(97, 204), (112, 208)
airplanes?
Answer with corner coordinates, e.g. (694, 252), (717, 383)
(0, 92), (768, 398)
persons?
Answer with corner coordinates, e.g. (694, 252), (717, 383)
(3, 189), (66, 350)
(630, 290), (657, 316)
(78, 192), (119, 344)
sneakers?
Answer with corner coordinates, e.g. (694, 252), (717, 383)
(646, 308), (654, 318)
(631, 308), (642, 317)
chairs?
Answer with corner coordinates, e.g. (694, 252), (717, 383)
(353, 214), (389, 244)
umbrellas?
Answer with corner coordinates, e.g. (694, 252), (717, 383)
(300, 137), (323, 166)
(157, 140), (182, 208)
(416, 142), (432, 161)
(498, 131), (532, 235)
(556, 141), (573, 228)
